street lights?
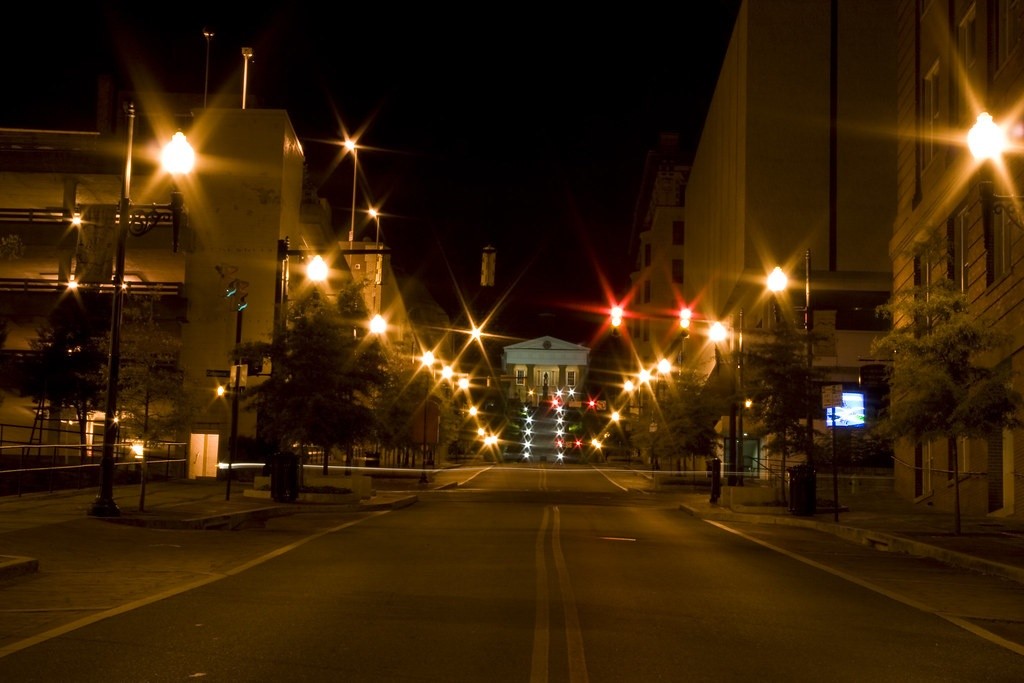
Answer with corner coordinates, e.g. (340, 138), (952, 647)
(708, 309), (745, 488)
(344, 139), (357, 250)
(402, 340), (436, 484)
(224, 277), (248, 501)
(767, 248), (815, 467)
(202, 30), (215, 109)
(84, 102), (196, 516)
(264, 233), (329, 476)
(240, 47), (254, 109)
(368, 208), (380, 246)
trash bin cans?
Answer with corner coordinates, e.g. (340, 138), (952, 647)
(787, 466), (818, 517)
(271, 451), (299, 502)
(364, 451), (381, 467)
